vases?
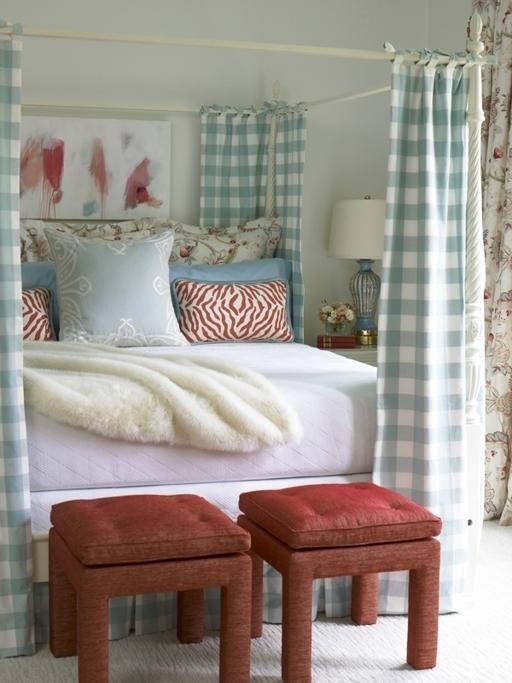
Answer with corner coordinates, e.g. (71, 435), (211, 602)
(326, 321), (348, 334)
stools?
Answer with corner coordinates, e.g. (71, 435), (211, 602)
(233, 480), (444, 682)
(45, 493), (256, 681)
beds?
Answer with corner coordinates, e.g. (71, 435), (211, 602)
(18, 218), (378, 644)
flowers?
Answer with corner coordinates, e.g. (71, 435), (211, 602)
(316, 298), (355, 324)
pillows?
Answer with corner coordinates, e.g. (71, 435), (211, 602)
(135, 216), (284, 267)
(20, 260), (59, 325)
(22, 285), (57, 342)
(20, 218), (139, 263)
(42, 226), (192, 348)
(171, 277), (296, 344)
(170, 257), (293, 328)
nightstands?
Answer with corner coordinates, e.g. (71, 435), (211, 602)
(327, 346), (378, 368)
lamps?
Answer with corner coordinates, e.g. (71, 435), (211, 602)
(326, 194), (385, 333)
